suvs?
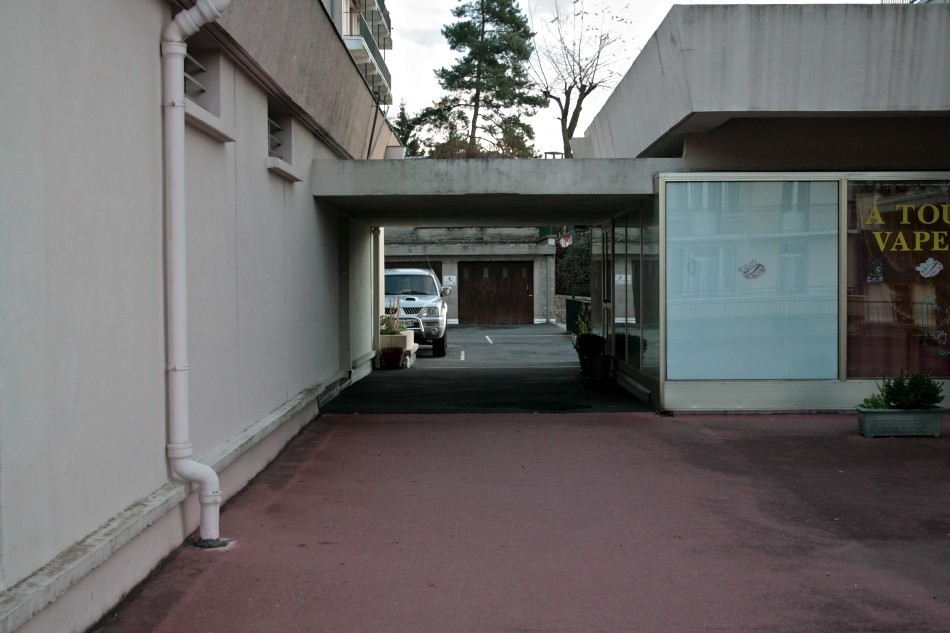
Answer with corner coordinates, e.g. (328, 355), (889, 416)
(383, 267), (451, 357)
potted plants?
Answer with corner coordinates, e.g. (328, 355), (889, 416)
(574, 333), (610, 383)
(856, 369), (945, 437)
(380, 296), (419, 368)
